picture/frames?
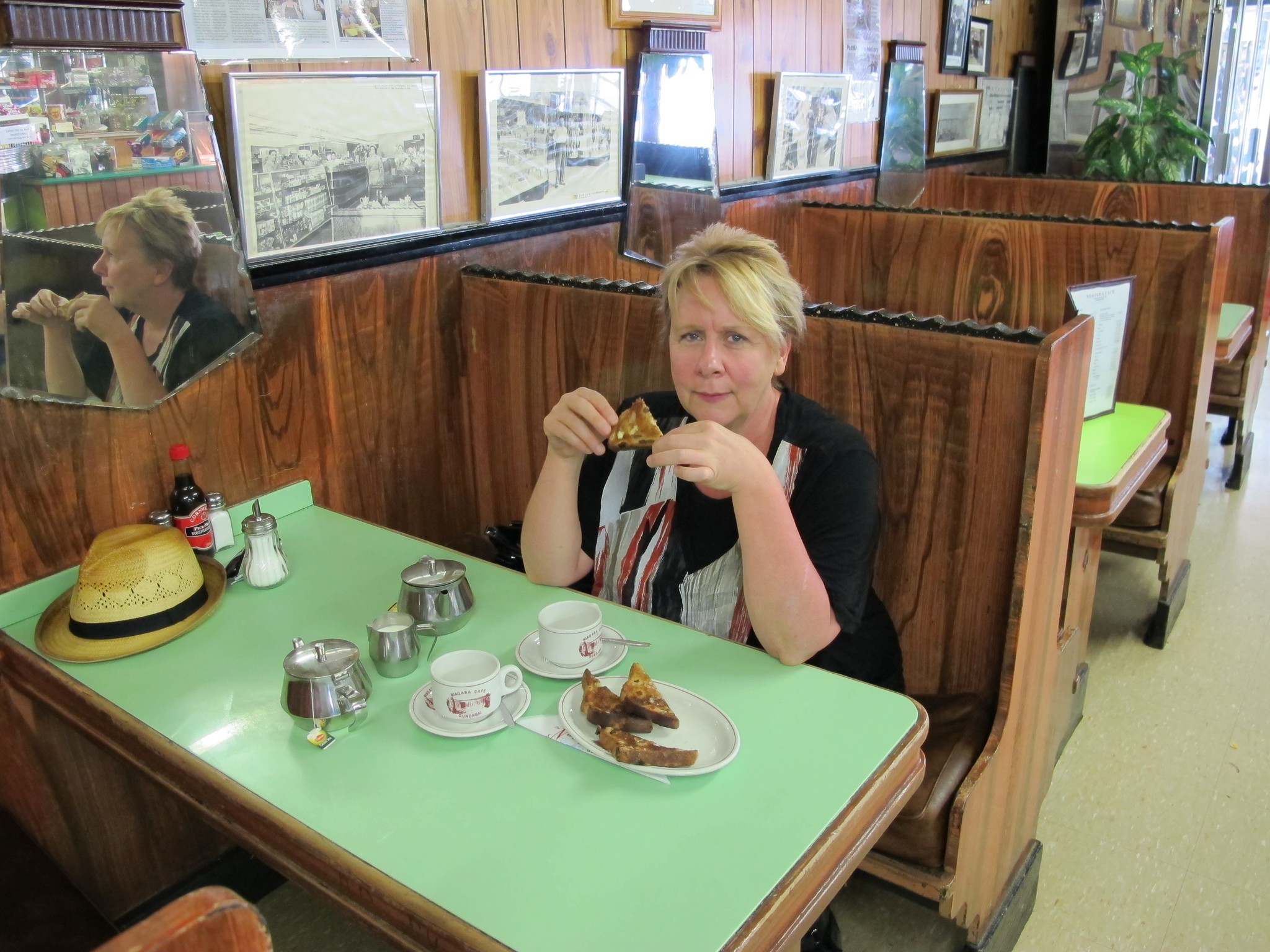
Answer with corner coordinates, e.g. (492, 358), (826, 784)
(1080, 13), (1106, 76)
(220, 72), (442, 264)
(1108, 0), (1145, 30)
(608, 0), (723, 32)
(1059, 31), (1087, 80)
(964, 15), (993, 76)
(941, 0), (971, 74)
(765, 71), (853, 180)
(476, 69), (623, 223)
(977, 77), (1014, 152)
(929, 89), (983, 157)
(1064, 84), (1103, 145)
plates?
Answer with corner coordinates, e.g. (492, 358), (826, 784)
(515, 624), (628, 679)
(409, 672), (531, 737)
(557, 676), (741, 776)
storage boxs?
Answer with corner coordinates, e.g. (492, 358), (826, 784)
(132, 110), (187, 149)
(132, 156), (174, 169)
(9, 67), (90, 89)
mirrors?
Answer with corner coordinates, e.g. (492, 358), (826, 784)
(0, 48), (265, 412)
(1047, 0), (1210, 175)
(874, 61), (927, 208)
(618, 50), (722, 268)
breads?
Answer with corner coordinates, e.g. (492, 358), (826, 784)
(608, 397), (663, 452)
(57, 291), (89, 323)
(580, 668), (653, 733)
(620, 662), (680, 729)
(595, 725), (698, 767)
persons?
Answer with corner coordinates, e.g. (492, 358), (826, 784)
(552, 115), (568, 189)
(365, 143), (384, 199)
(394, 145), (408, 169)
(969, 29), (983, 65)
(282, 0), (325, 20)
(343, 150), (354, 164)
(263, 150), (283, 172)
(340, 2), (366, 37)
(520, 221), (905, 952)
(12, 187), (246, 408)
(323, 152), (341, 166)
(365, 152), (371, 162)
(793, 90), (836, 169)
(361, 3), (378, 31)
(947, 4), (966, 56)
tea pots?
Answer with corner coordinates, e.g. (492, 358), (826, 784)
(397, 555), (476, 637)
(279, 636), (374, 732)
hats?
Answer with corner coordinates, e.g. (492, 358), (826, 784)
(35, 524), (227, 664)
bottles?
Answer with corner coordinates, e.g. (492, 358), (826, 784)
(148, 509), (174, 526)
(205, 491), (235, 553)
(241, 498), (290, 590)
(168, 444), (215, 559)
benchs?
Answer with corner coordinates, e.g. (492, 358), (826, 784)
(0, 172), (1270, 952)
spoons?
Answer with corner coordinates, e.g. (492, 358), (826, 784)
(500, 695), (516, 729)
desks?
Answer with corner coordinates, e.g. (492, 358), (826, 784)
(0, 507), (932, 952)
(1062, 401), (1172, 664)
(1214, 303), (1254, 366)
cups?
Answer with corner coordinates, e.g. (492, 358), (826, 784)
(538, 600), (603, 669)
(430, 649), (523, 724)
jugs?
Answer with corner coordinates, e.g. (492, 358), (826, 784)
(365, 612), (439, 678)
